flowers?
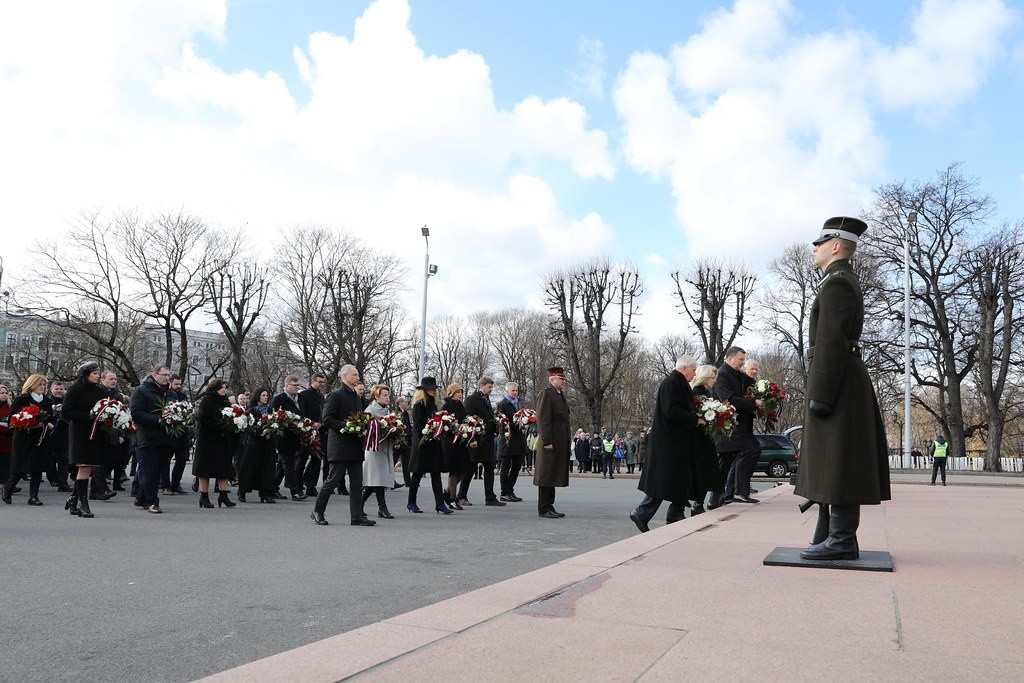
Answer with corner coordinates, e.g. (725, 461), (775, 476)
(689, 394), (740, 445)
(378, 412), (409, 446)
(150, 397), (195, 438)
(219, 404), (326, 459)
(417, 410), (461, 450)
(452, 414), (486, 450)
(339, 411), (375, 437)
(88, 396), (139, 440)
(0, 404), (63, 446)
(513, 410), (537, 432)
(490, 410), (513, 449)
(744, 379), (786, 434)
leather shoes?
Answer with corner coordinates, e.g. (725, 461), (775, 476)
(537, 508), (565, 519)
(0, 477), (523, 525)
(733, 493), (760, 503)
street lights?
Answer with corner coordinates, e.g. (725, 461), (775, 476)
(904, 211), (918, 468)
(418, 225), (438, 385)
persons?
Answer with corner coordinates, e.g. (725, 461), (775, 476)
(192, 373), (327, 508)
(792, 216), (891, 561)
(911, 448), (924, 468)
(630, 355), (726, 533)
(0, 362), (138, 518)
(406, 377), (528, 514)
(310, 364), (420, 526)
(128, 364), (189, 513)
(707, 347), (759, 511)
(929, 434), (949, 486)
(473, 427), (539, 479)
(533, 366), (570, 518)
(569, 427), (652, 479)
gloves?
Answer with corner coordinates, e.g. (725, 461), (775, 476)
(810, 398), (831, 418)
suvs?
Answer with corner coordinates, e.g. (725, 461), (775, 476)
(753, 433), (799, 479)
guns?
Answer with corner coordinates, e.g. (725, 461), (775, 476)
(798, 500), (830, 544)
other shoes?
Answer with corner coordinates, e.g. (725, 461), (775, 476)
(578, 467), (642, 479)
(749, 487), (758, 493)
(666, 515), (684, 525)
(930, 482), (935, 486)
(630, 508), (650, 533)
(942, 481), (946, 486)
(706, 500), (718, 510)
(691, 507), (703, 516)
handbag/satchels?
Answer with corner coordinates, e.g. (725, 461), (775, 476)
(631, 444), (637, 454)
(617, 446), (625, 459)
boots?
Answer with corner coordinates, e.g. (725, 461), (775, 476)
(798, 498), (859, 560)
(479, 465), (483, 479)
(473, 466), (478, 479)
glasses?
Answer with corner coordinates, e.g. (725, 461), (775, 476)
(289, 383), (300, 387)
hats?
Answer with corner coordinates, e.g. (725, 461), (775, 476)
(626, 431), (633, 436)
(546, 366), (567, 379)
(812, 217), (868, 246)
(416, 377), (443, 390)
(75, 361), (99, 378)
(530, 428), (538, 433)
(446, 383), (464, 398)
